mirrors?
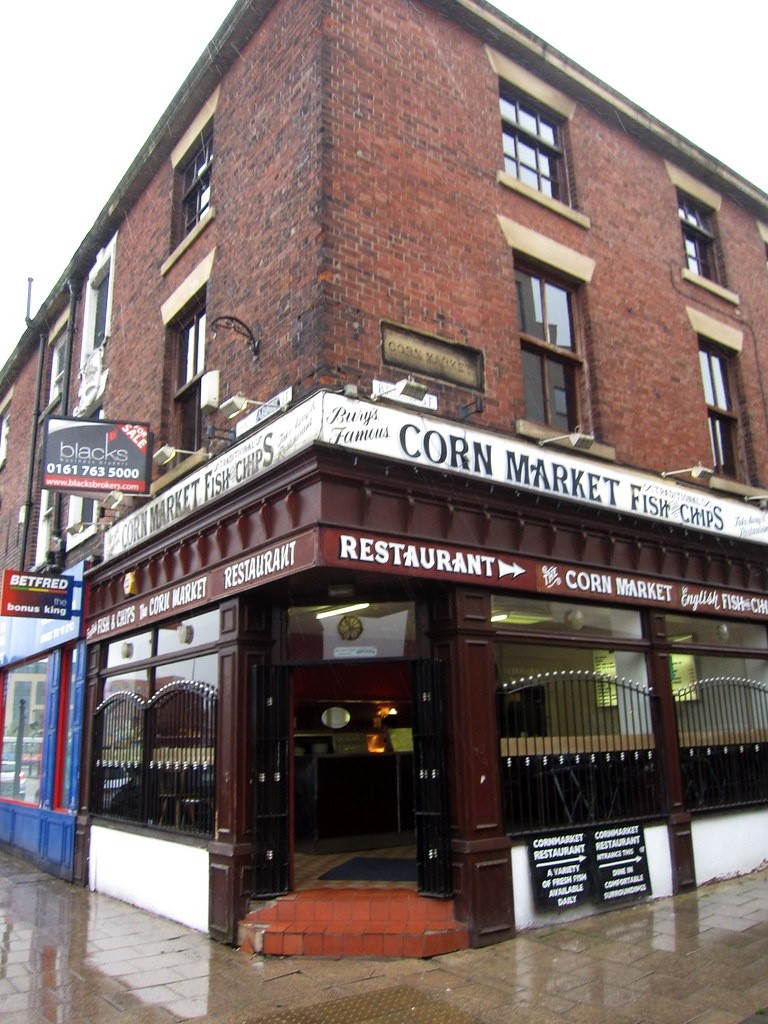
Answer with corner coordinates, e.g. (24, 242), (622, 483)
(320, 707), (351, 731)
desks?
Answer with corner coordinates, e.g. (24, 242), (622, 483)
(157, 795), (214, 830)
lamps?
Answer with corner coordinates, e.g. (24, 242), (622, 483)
(540, 425), (594, 450)
(100, 486), (156, 510)
(153, 444), (217, 466)
(662, 461), (714, 482)
(65, 522), (114, 535)
(370, 375), (428, 403)
(459, 394), (487, 422)
(218, 392), (288, 420)
(206, 424), (234, 443)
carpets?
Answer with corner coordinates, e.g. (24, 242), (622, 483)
(317, 855), (417, 881)
